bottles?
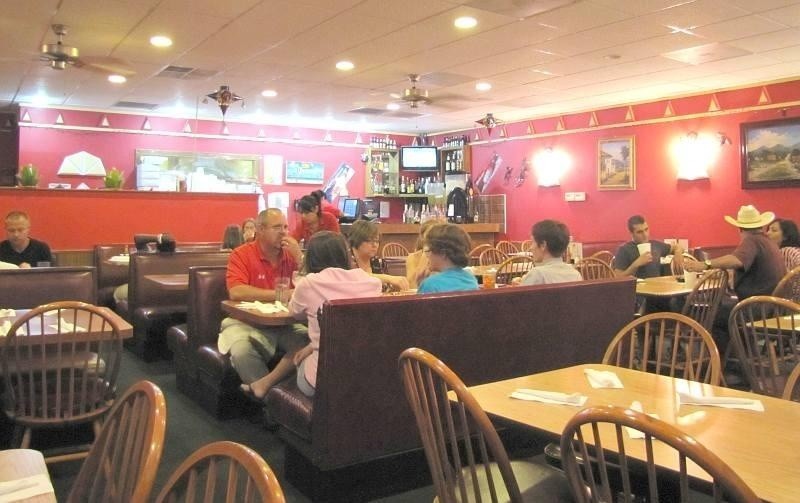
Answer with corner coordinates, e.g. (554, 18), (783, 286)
(445, 150), (464, 173)
(402, 203), (447, 225)
(370, 137), (398, 149)
(399, 171), (440, 194)
(442, 135), (465, 146)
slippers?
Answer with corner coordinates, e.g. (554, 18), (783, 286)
(237, 383), (265, 405)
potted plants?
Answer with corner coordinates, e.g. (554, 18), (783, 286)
(14, 164), (42, 189)
(105, 166), (128, 190)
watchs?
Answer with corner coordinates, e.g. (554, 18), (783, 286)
(704, 259), (712, 269)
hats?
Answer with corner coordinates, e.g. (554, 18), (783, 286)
(723, 204), (776, 229)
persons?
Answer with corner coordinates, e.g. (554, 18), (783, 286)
(682, 204), (787, 376)
(765, 217), (800, 319)
(0, 211), (52, 268)
(615, 214), (686, 361)
(112, 230), (175, 301)
(519, 218), (583, 286)
(215, 189), (479, 431)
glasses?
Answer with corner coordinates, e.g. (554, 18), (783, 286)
(364, 238), (382, 246)
(271, 226), (289, 234)
(421, 249), (433, 257)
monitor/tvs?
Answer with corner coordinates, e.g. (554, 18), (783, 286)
(341, 198), (367, 220)
(399, 146), (440, 171)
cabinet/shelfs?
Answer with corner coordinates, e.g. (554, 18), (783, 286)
(363, 145), (401, 200)
(440, 144), (473, 193)
(399, 168), (442, 199)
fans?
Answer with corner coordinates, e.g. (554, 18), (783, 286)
(357, 72), (477, 113)
(0, 23), (139, 81)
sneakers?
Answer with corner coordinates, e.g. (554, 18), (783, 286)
(262, 408), (280, 431)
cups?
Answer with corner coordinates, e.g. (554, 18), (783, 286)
(636, 243), (652, 259)
(276, 276), (290, 307)
(482, 270), (497, 288)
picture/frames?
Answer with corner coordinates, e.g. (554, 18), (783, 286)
(738, 114), (800, 192)
(595, 134), (636, 193)
(285, 158), (324, 186)
